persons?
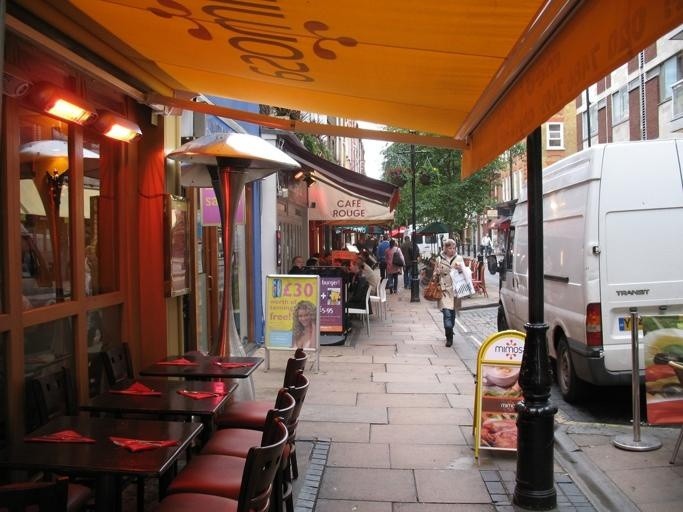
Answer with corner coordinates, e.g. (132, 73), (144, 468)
(384, 238), (405, 294)
(288, 256), (308, 274)
(342, 257), (369, 336)
(401, 235), (417, 289)
(305, 257), (319, 267)
(376, 236), (389, 284)
(432, 239), (466, 348)
(481, 232), (493, 259)
(289, 299), (317, 351)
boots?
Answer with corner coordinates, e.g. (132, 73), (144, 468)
(390, 286), (393, 293)
(445, 326), (454, 346)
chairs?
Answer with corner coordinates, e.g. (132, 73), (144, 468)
(199, 369), (310, 478)
(167, 388), (296, 511)
(216, 348), (308, 427)
(101, 342), (133, 383)
(374, 269), (380, 286)
(155, 417), (288, 512)
(379, 279), (388, 320)
(370, 277), (380, 320)
(0, 476), (91, 512)
(22, 379), (49, 434)
(344, 285), (372, 337)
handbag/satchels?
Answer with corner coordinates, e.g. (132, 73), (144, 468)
(424, 275), (442, 301)
(450, 268), (475, 299)
(393, 247), (403, 266)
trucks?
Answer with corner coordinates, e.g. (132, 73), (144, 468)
(19, 222), (71, 337)
(486, 137), (683, 402)
(402, 223), (439, 259)
